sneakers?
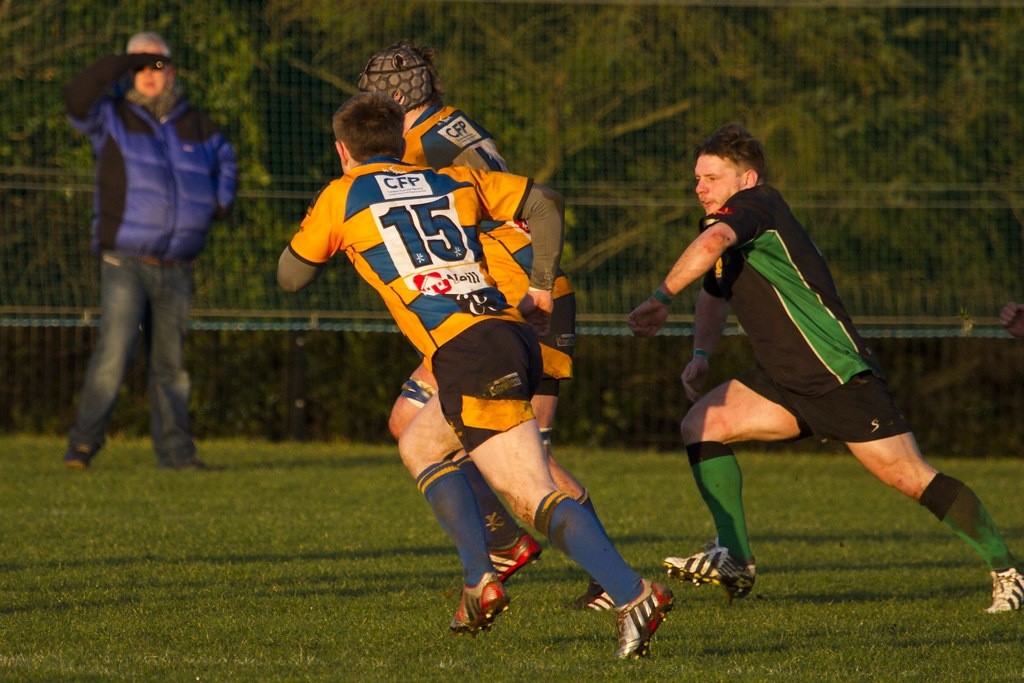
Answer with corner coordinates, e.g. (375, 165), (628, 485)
(450, 571), (509, 638)
(612, 579), (675, 660)
(158, 456), (223, 472)
(488, 526), (541, 583)
(981, 567), (1024, 615)
(63, 441), (96, 468)
(572, 577), (616, 612)
(663, 546), (756, 598)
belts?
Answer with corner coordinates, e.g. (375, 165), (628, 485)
(137, 254), (174, 266)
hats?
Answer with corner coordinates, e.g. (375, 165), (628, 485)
(356, 45), (434, 116)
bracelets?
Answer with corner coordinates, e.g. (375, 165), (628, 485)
(693, 348), (709, 359)
(650, 289), (672, 306)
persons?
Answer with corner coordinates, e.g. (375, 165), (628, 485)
(274, 93), (674, 660)
(355, 47), (624, 612)
(58, 32), (242, 472)
(623, 123), (1024, 614)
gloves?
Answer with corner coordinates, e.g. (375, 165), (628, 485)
(128, 54), (172, 69)
